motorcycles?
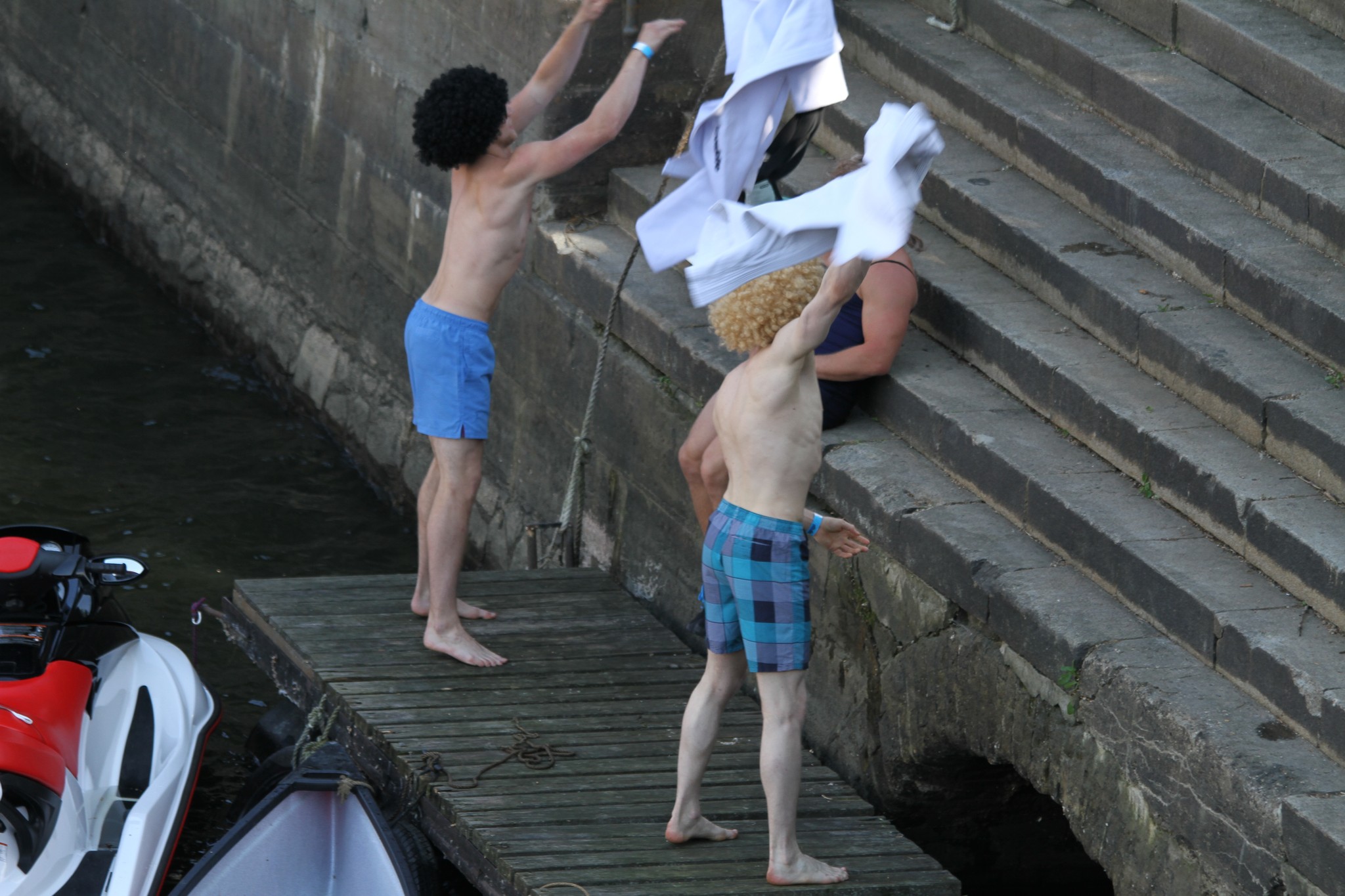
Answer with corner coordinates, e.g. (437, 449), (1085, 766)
(0, 521), (221, 896)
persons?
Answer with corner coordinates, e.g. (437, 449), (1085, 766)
(700, 243), (917, 511)
(403, 1), (688, 668)
(664, 251), (871, 884)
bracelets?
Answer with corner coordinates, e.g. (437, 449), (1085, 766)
(804, 513), (823, 537)
(632, 43), (654, 60)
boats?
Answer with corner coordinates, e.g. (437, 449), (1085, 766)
(171, 739), (425, 896)
